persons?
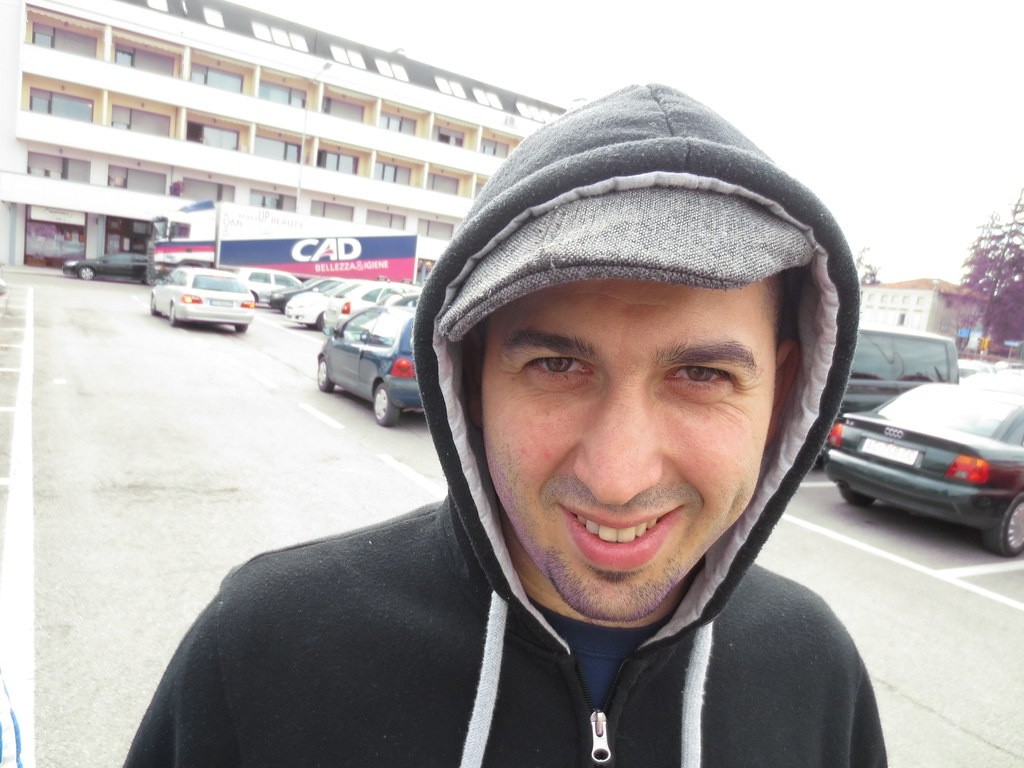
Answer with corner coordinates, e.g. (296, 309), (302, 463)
(122, 81), (889, 768)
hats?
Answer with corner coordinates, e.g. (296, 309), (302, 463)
(439, 187), (816, 344)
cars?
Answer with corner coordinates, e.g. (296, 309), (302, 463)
(233, 266), (302, 303)
(284, 280), (368, 331)
(268, 277), (341, 314)
(957, 360), (1024, 382)
(315, 306), (425, 428)
(149, 267), (256, 332)
(62, 252), (156, 286)
(376, 288), (423, 308)
(823, 379), (1024, 558)
(322, 282), (410, 331)
(812, 322), (961, 470)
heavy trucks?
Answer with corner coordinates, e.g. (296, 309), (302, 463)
(146, 200), (420, 283)
